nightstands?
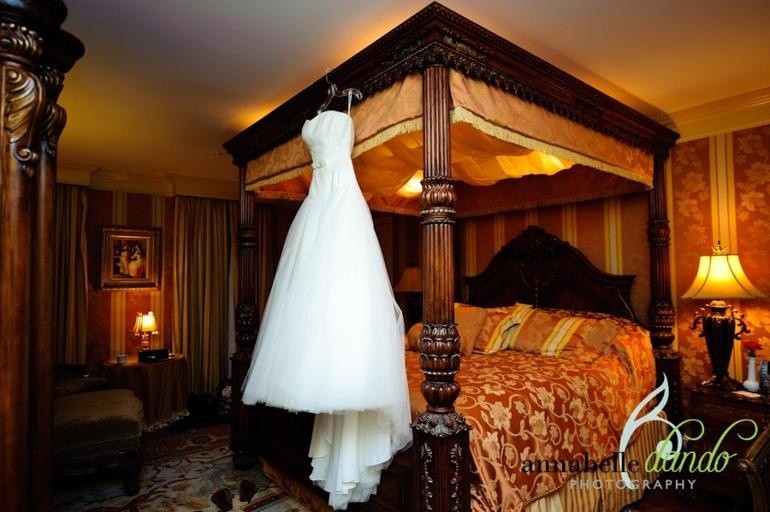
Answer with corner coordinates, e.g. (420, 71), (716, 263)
(678, 386), (770, 511)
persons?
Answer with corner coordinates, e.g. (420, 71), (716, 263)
(120, 245), (129, 276)
(129, 245), (145, 278)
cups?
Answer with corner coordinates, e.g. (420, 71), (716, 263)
(116, 354), (126, 365)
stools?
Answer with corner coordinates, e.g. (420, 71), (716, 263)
(50, 375), (145, 495)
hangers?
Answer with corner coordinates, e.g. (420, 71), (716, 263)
(317, 66), (363, 114)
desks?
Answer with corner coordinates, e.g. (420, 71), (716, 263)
(96, 352), (190, 452)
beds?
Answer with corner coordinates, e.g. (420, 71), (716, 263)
(223, 2), (681, 512)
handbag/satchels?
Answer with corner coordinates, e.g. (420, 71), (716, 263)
(187, 379), (232, 427)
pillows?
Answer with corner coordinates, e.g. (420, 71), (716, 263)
(406, 302), (617, 363)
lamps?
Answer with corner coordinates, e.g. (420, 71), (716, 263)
(679, 245), (768, 389)
(133, 311), (159, 352)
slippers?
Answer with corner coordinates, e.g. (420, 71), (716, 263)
(238, 480), (256, 502)
(212, 487), (233, 510)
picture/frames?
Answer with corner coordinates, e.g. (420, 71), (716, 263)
(97, 225), (162, 293)
